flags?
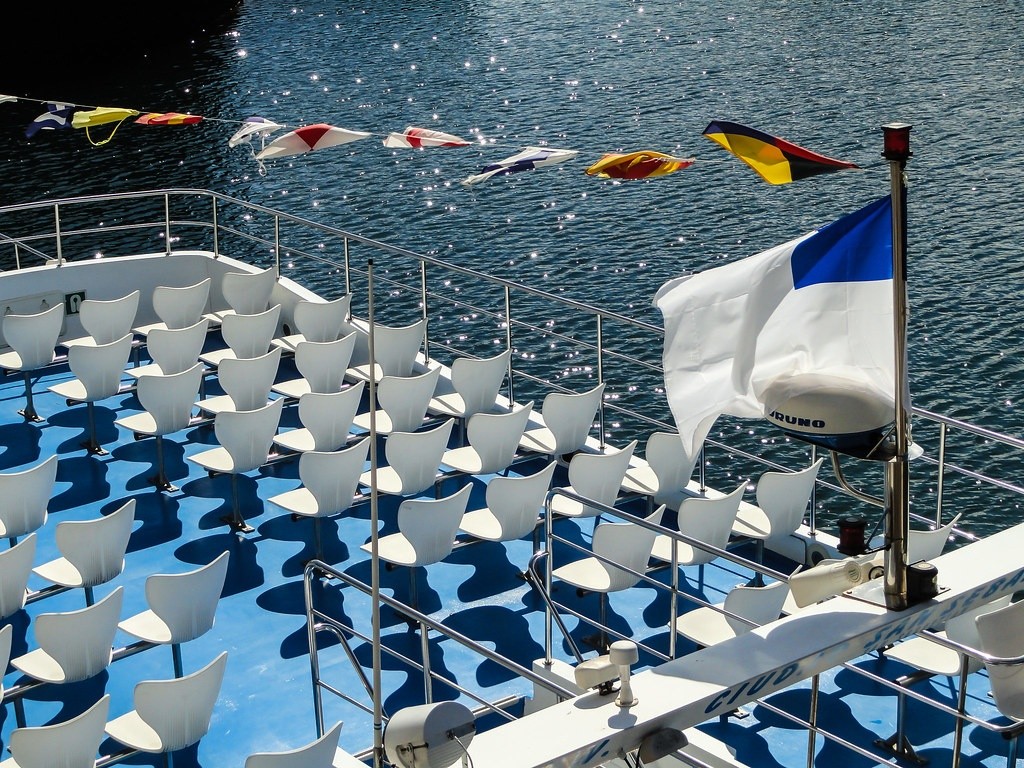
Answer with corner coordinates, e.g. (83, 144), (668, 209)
(228, 117), (286, 148)
(382, 126), (475, 148)
(652, 186), (912, 460)
(248, 123), (373, 160)
(0, 94), (17, 103)
(24, 101), (75, 138)
(584, 150), (696, 179)
(71, 106), (139, 129)
(702, 120), (862, 185)
(133, 112), (204, 125)
(461, 146), (579, 185)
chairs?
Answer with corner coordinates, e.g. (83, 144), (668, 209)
(0, 267), (1024, 768)
